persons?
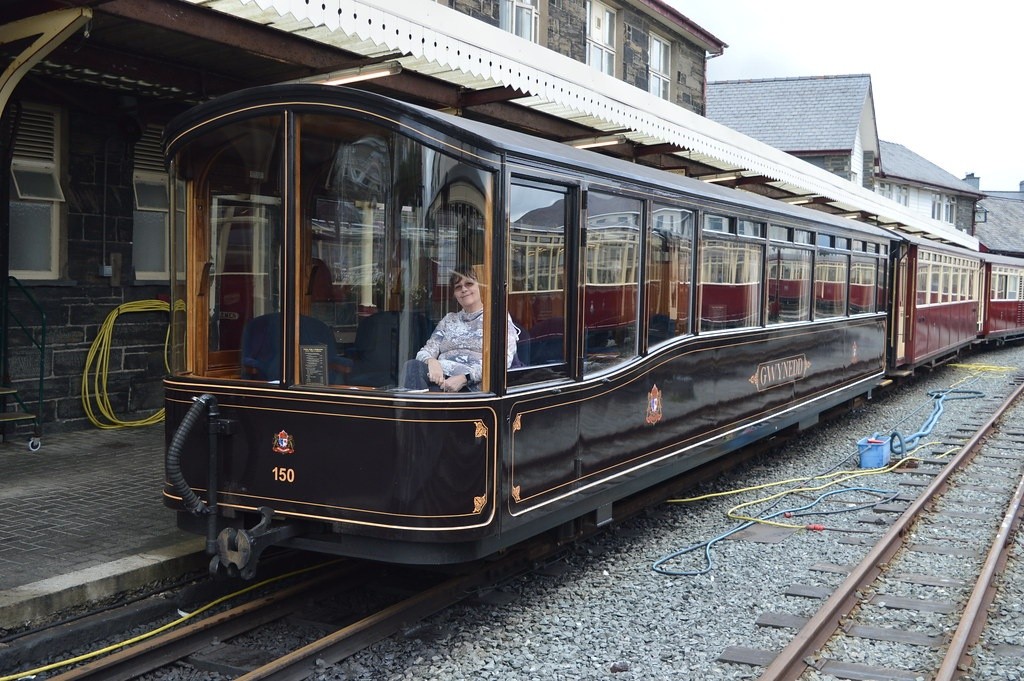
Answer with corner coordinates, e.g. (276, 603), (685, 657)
(401, 266), (521, 392)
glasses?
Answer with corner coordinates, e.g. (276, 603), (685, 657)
(454, 282), (473, 291)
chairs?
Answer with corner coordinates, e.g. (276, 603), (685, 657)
(515, 322), (531, 365)
(649, 314), (674, 347)
(586, 309), (625, 353)
(528, 318), (588, 366)
(344, 310), (435, 387)
(241, 313), (346, 385)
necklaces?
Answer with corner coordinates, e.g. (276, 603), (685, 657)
(462, 313), (482, 322)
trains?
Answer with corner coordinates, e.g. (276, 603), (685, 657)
(158, 83), (1024, 562)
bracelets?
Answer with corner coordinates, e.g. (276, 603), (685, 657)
(465, 372), (471, 385)
